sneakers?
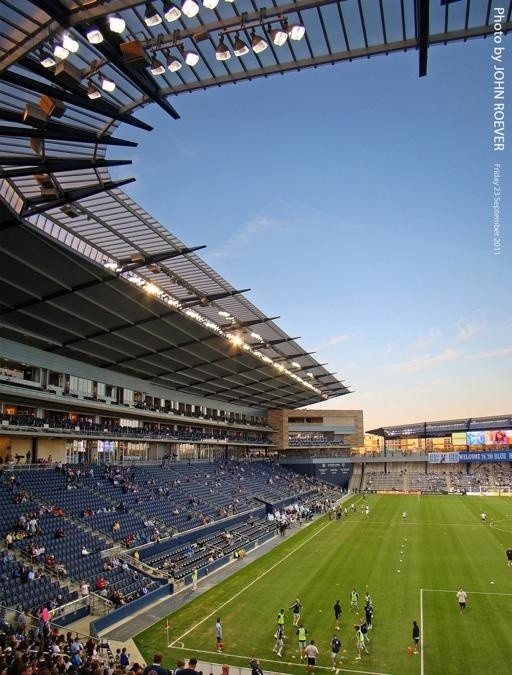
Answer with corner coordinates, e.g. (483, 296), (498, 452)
(354, 654), (361, 660)
(292, 621), (299, 627)
(300, 654), (307, 661)
(412, 650), (419, 655)
(271, 648), (282, 657)
(330, 666), (336, 672)
(273, 633), (286, 638)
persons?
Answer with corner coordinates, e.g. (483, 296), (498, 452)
(455, 587), (468, 614)
(360, 617), (370, 645)
(353, 626), (370, 661)
(492, 432), (509, 445)
(360, 447), (512, 495)
(361, 495), (366, 502)
(479, 511), (486, 524)
(505, 547), (512, 567)
(364, 504), (369, 516)
(0, 412), (360, 675)
(401, 512), (407, 520)
(363, 600), (374, 630)
(364, 592), (373, 619)
(360, 503), (365, 514)
(411, 621), (421, 655)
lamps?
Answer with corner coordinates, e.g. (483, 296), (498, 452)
(39, 0), (307, 100)
